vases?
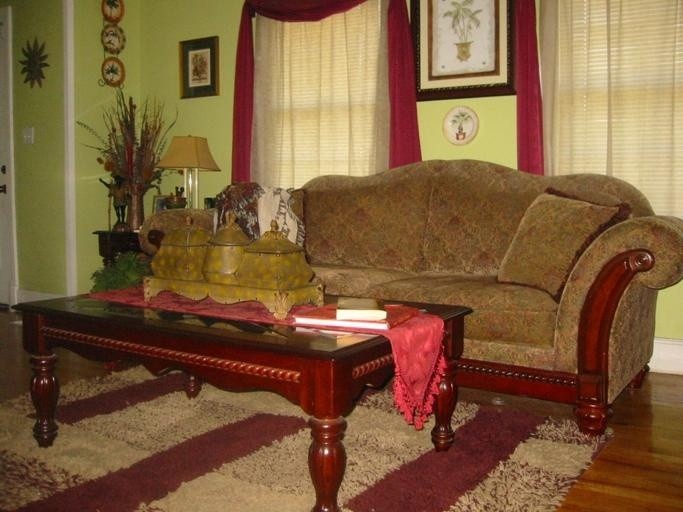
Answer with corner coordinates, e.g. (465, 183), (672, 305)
(121, 184), (142, 230)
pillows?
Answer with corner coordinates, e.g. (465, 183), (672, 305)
(494, 188), (635, 306)
(244, 187), (310, 265)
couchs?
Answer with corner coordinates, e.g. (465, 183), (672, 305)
(138, 156), (683, 435)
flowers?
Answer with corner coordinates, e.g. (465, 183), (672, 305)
(74, 86), (181, 189)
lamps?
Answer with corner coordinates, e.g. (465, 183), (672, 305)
(155, 130), (220, 212)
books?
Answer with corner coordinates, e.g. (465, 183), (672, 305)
(335, 296), (388, 322)
(288, 303), (420, 332)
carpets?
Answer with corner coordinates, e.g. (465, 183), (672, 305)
(0, 366), (617, 512)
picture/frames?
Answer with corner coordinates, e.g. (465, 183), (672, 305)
(426, 0), (501, 82)
(177, 34), (218, 102)
